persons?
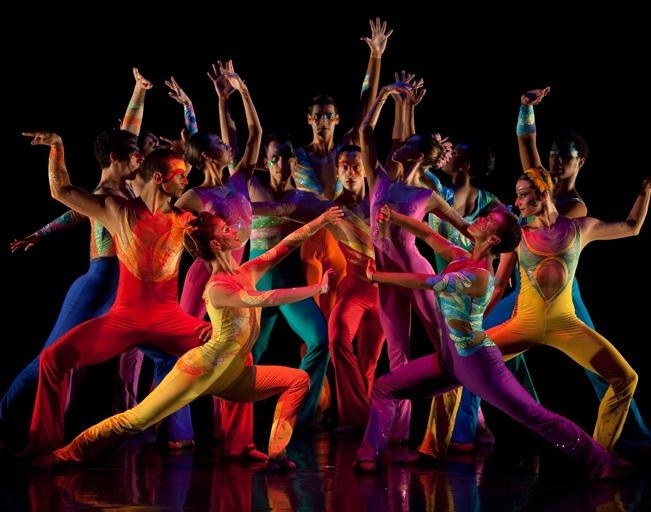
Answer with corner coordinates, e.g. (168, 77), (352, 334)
(2, 14), (651, 480)
